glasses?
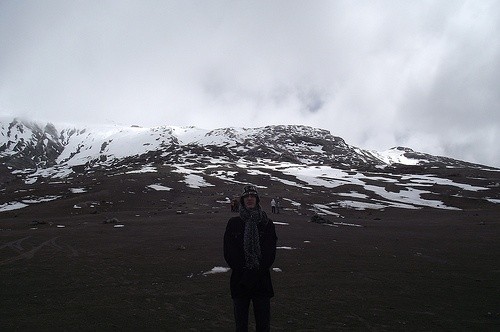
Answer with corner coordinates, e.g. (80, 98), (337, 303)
(243, 195), (257, 198)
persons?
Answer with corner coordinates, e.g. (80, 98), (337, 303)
(230, 196), (281, 214)
(222, 184), (278, 332)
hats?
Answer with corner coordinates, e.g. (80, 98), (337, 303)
(240, 185), (260, 202)
